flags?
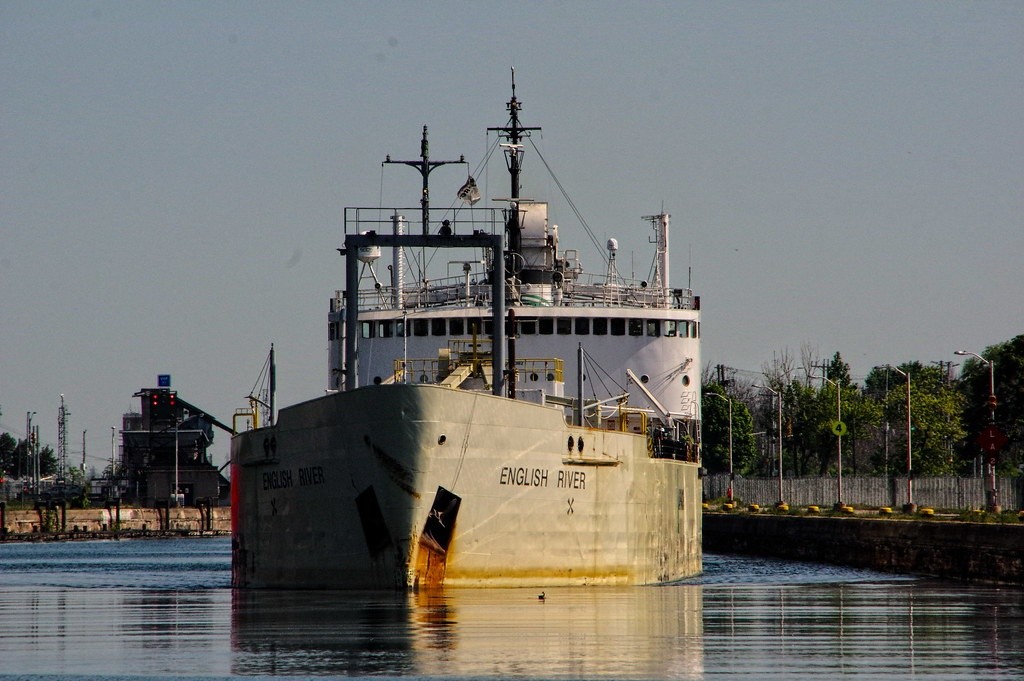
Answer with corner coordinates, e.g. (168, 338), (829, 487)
(457, 176), (480, 206)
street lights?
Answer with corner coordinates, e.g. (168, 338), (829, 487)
(807, 374), (846, 510)
(952, 350), (1003, 514)
(888, 364), (918, 514)
(751, 384), (787, 508)
(704, 392), (738, 509)
(173, 412), (206, 507)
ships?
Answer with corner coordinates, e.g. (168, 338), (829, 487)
(233, 64), (704, 604)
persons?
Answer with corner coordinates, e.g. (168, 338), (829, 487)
(650, 425), (674, 459)
